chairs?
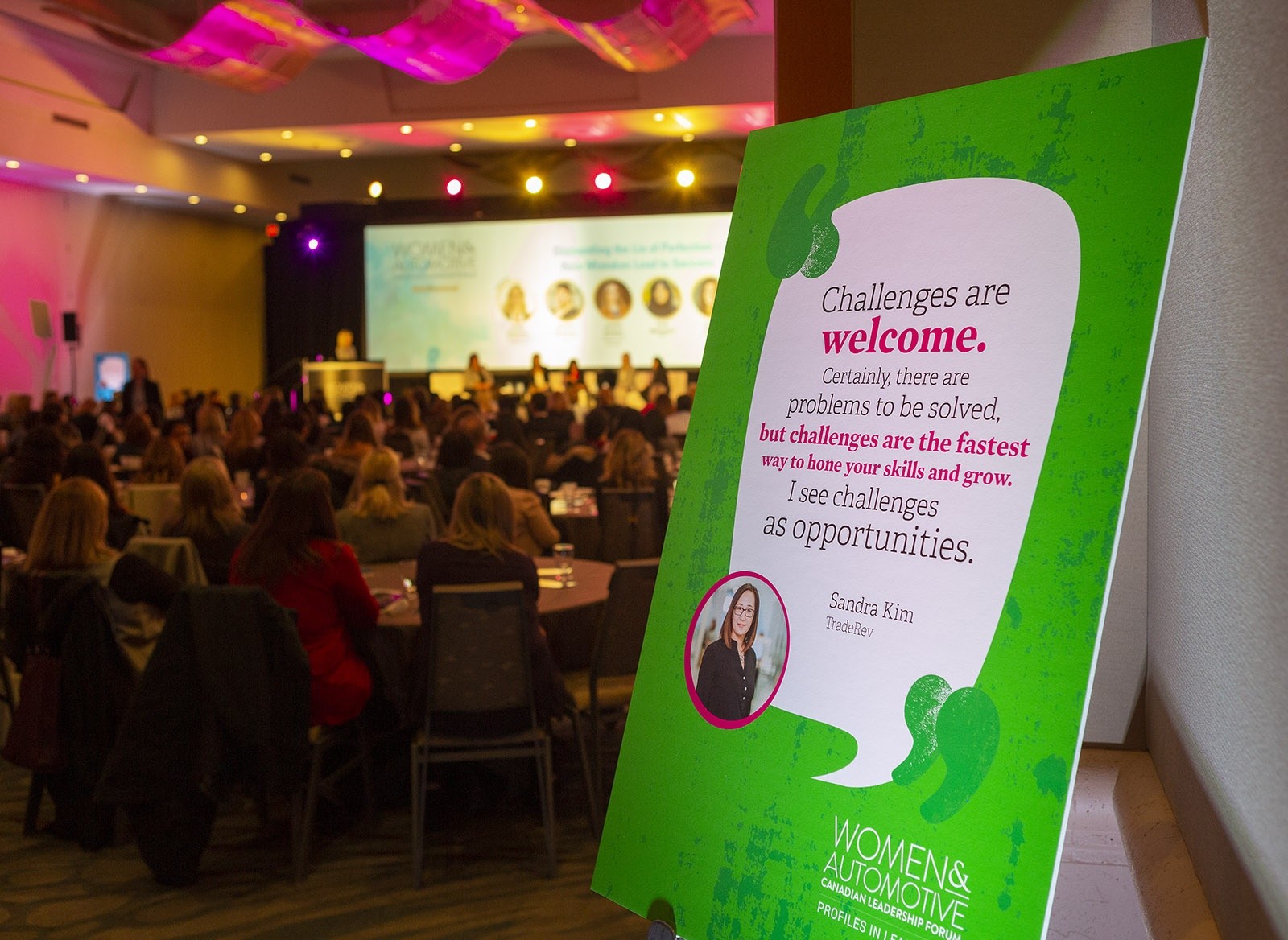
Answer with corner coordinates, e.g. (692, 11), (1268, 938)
(409, 583), (559, 887)
(0, 484), (43, 550)
(595, 486), (655, 563)
(557, 561), (661, 840)
(1, 565), (130, 854)
(131, 588), (374, 876)
(131, 536), (209, 588)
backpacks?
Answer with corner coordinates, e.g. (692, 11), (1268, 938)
(0, 572), (94, 774)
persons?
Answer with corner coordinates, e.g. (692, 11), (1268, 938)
(504, 280), (720, 321)
(694, 584), (765, 720)
(0, 329), (701, 831)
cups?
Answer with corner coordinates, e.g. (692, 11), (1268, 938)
(533, 476), (596, 514)
(234, 470), (250, 488)
(552, 544), (574, 586)
(414, 449), (429, 471)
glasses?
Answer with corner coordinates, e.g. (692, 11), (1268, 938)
(733, 606), (756, 618)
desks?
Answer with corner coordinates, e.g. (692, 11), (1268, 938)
(104, 483), (184, 535)
(357, 558), (616, 740)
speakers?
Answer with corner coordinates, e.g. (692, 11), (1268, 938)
(65, 314), (77, 341)
